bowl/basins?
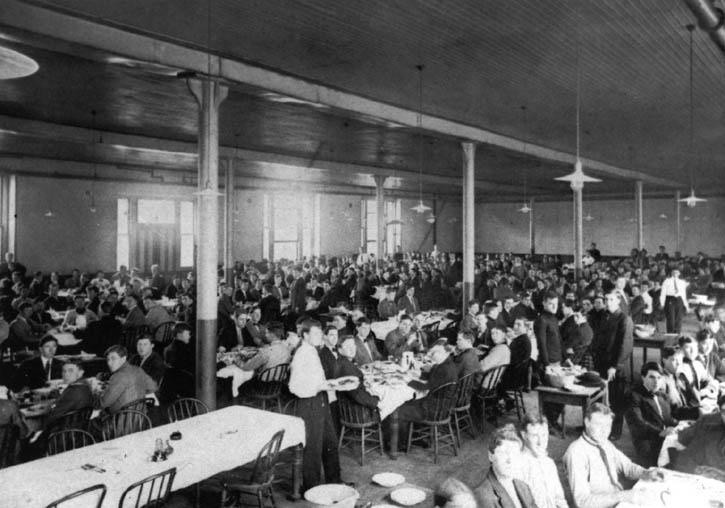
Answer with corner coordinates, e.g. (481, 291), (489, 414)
(302, 483), (360, 508)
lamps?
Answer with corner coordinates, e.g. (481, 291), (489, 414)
(410, 30), (604, 215)
(676, 23), (707, 209)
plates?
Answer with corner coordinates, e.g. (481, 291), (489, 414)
(390, 487), (427, 504)
(368, 472), (405, 488)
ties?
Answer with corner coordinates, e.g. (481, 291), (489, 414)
(45, 358), (50, 379)
(597, 445), (623, 491)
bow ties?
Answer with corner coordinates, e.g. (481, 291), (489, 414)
(648, 389), (660, 399)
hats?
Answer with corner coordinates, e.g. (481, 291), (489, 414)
(576, 372), (606, 387)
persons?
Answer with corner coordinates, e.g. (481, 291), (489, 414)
(2, 242), (725, 466)
(561, 401), (668, 507)
(673, 398), (725, 483)
(520, 412), (570, 507)
(289, 317), (355, 500)
(472, 421), (538, 508)
(433, 476), (478, 508)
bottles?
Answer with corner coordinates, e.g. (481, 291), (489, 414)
(150, 439), (173, 462)
